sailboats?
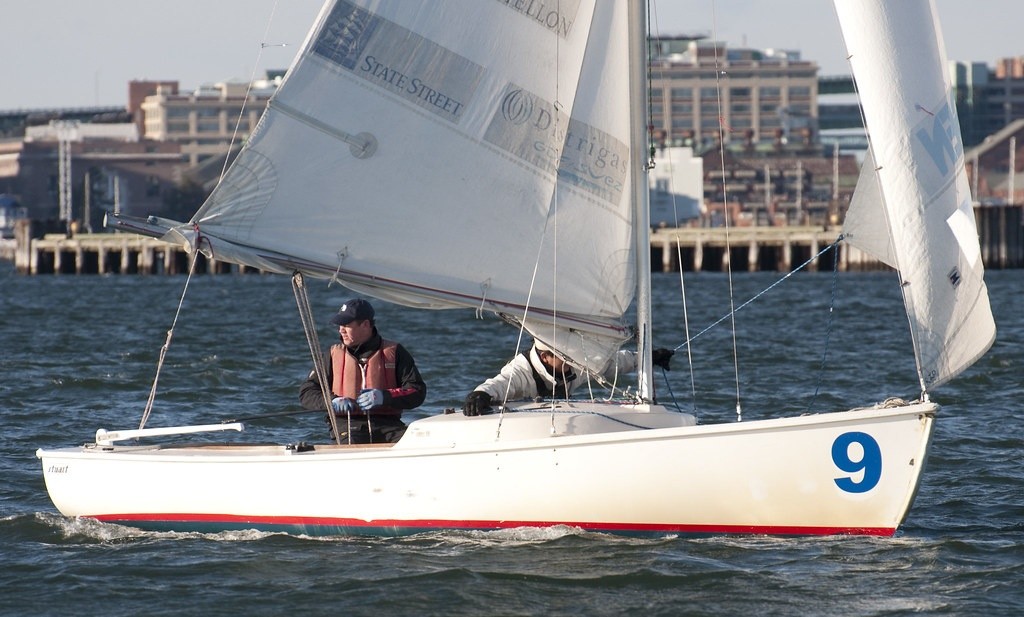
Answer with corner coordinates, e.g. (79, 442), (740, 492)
(36, 0), (997, 538)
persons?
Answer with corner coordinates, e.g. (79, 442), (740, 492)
(298, 299), (426, 444)
(442, 338), (676, 417)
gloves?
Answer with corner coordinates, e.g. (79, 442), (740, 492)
(462, 390), (491, 416)
(332, 396), (355, 416)
(651, 348), (674, 371)
(355, 388), (384, 410)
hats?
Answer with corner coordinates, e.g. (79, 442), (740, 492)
(534, 335), (550, 351)
(330, 298), (374, 325)
